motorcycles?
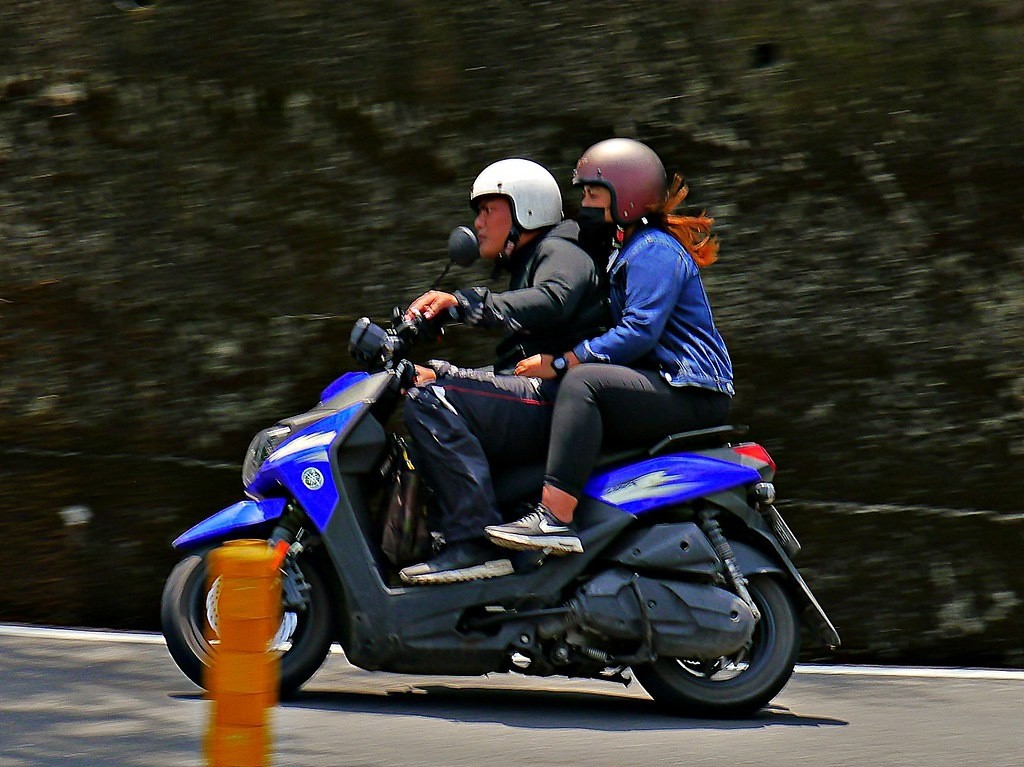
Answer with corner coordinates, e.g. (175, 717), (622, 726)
(156, 225), (844, 723)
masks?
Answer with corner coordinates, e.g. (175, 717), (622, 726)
(575, 206), (616, 233)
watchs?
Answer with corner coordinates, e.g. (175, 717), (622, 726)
(551, 353), (569, 375)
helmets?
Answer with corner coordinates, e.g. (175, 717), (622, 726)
(573, 137), (665, 227)
(468, 159), (563, 233)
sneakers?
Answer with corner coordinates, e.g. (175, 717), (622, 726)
(398, 537), (515, 584)
(483, 501), (584, 557)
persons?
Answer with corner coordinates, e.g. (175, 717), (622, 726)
(483, 138), (735, 557)
(400, 159), (618, 582)
